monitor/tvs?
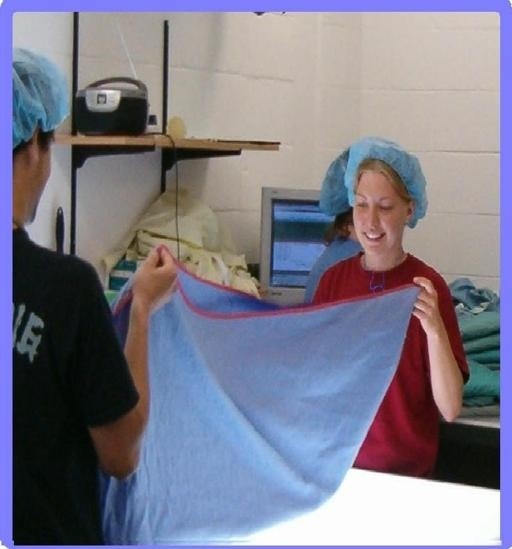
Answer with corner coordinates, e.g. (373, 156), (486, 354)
(259, 187), (338, 306)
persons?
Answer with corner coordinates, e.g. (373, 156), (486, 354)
(304, 146), (363, 307)
(310, 138), (469, 479)
(12, 45), (179, 545)
